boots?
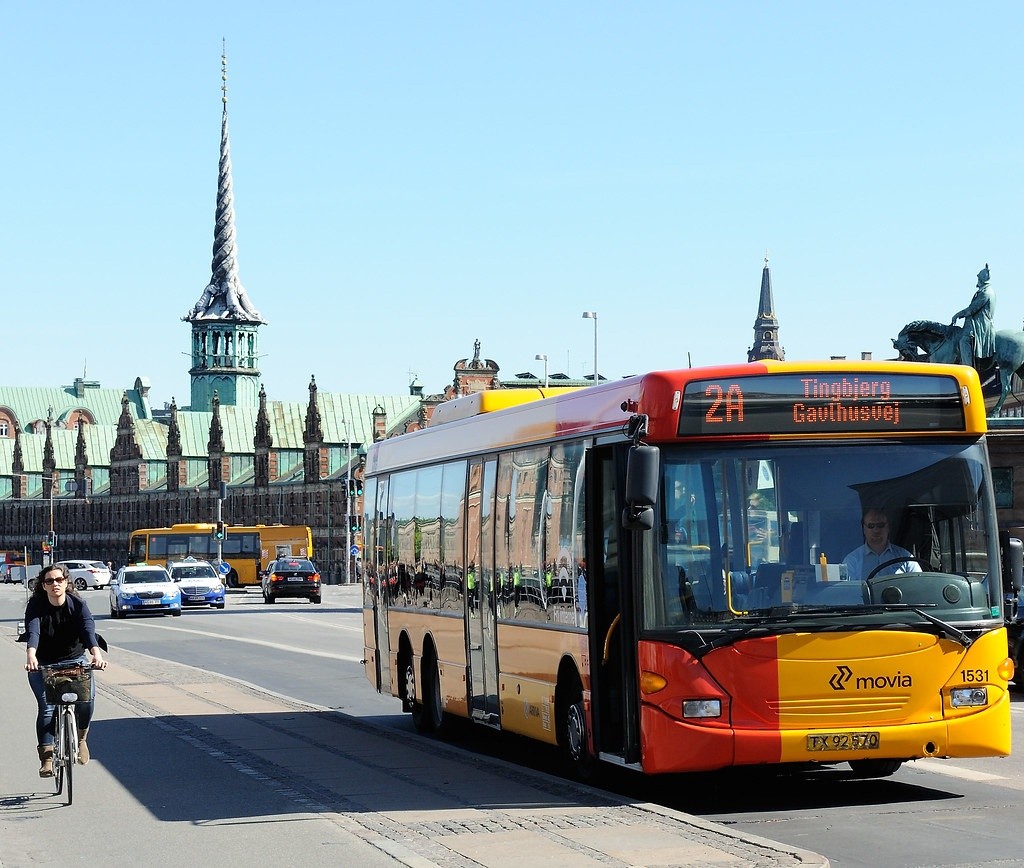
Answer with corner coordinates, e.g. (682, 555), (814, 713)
(78, 726), (90, 765)
(37, 745), (53, 772)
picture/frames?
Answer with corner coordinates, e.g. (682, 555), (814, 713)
(989, 466), (1014, 509)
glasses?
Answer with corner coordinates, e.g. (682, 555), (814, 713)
(43, 577), (65, 585)
(864, 521), (888, 529)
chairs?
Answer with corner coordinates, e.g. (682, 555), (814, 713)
(402, 540), (788, 613)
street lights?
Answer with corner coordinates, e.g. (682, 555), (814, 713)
(536, 355), (549, 389)
(581, 311), (598, 386)
(342, 419), (354, 583)
(194, 478), (222, 584)
(50, 477), (92, 564)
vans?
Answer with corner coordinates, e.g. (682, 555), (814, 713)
(0, 564), (25, 584)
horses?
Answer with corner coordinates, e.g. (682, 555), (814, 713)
(891, 320), (1024, 418)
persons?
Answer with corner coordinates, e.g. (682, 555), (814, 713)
(841, 509), (922, 581)
(367, 560), (587, 621)
(17, 564), (108, 773)
(747, 492), (779, 571)
(951, 267), (995, 366)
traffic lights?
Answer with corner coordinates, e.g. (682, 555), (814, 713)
(355, 480), (363, 498)
(48, 531), (54, 546)
(217, 522), (224, 540)
(357, 515), (364, 532)
(350, 515), (357, 532)
(348, 480), (355, 497)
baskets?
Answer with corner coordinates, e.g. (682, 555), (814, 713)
(43, 668), (92, 704)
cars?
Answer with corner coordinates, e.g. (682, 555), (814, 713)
(939, 551), (1024, 684)
(260, 558), (322, 605)
(24, 559), (111, 589)
(169, 557), (225, 609)
(109, 564), (182, 617)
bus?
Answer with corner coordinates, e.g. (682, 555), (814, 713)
(128, 523), (311, 587)
(362, 357), (1023, 781)
(718, 509), (799, 567)
